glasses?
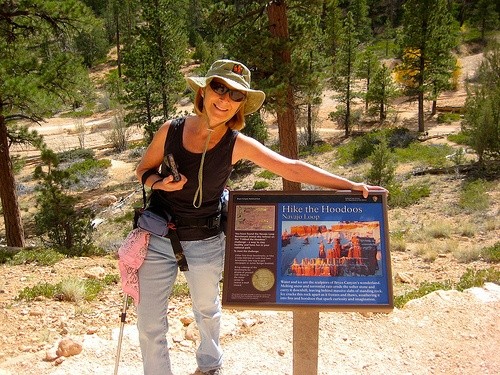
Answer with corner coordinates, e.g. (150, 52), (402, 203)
(210, 81), (246, 102)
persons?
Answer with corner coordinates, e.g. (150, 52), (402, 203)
(135, 60), (389, 375)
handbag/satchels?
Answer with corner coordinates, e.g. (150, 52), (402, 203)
(135, 207), (174, 238)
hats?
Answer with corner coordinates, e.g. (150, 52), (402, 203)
(186, 59), (266, 116)
